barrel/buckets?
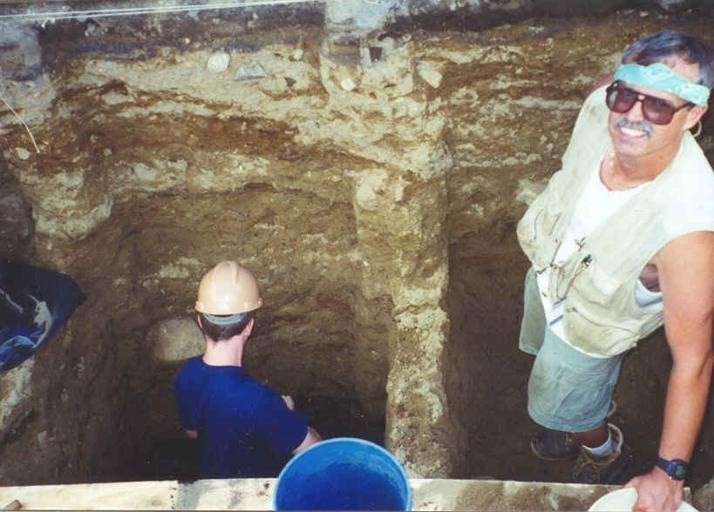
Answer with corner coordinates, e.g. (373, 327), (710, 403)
(272, 437), (411, 511)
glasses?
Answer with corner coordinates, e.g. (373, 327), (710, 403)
(606, 81), (691, 124)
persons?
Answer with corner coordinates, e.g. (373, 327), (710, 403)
(515, 30), (714, 512)
(175, 261), (322, 484)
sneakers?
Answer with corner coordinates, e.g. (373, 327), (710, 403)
(563, 424), (634, 483)
(531, 400), (617, 460)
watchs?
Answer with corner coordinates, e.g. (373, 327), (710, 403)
(655, 455), (691, 481)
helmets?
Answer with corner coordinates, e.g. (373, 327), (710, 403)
(194, 260), (263, 315)
